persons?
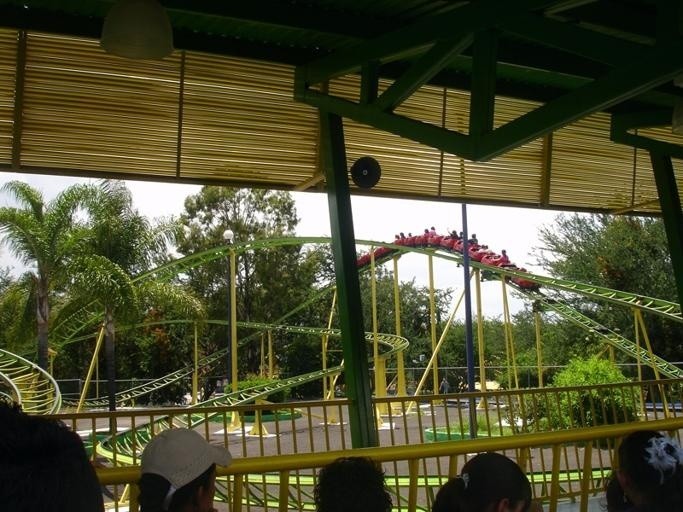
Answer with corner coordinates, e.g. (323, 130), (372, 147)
(314, 456), (393, 512)
(137, 427), (233, 512)
(616, 429), (683, 512)
(606, 475), (634, 512)
(431, 452), (532, 512)
(439, 378), (450, 394)
(394, 226), (508, 265)
(0, 396), (105, 512)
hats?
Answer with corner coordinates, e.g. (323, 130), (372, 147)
(141, 428), (232, 490)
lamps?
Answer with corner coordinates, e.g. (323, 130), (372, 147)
(96, 0), (175, 61)
